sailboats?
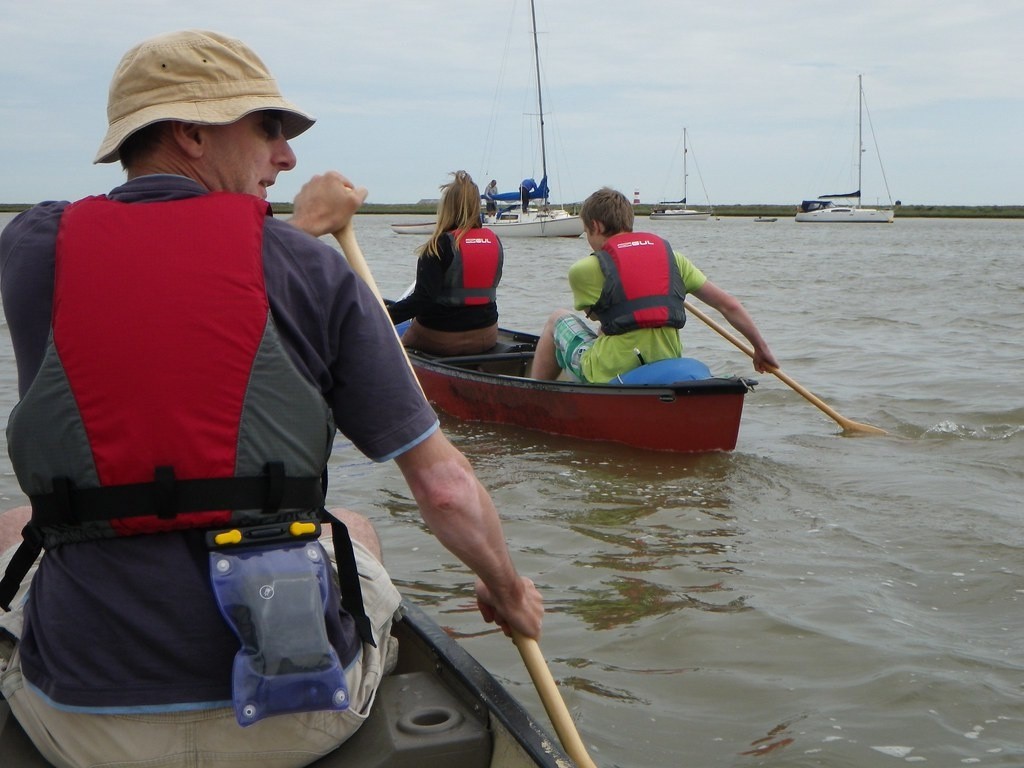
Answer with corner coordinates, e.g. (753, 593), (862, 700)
(795, 74), (896, 223)
(389, 0), (584, 235)
(649, 127), (722, 221)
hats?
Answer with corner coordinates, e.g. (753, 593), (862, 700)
(93, 29), (318, 165)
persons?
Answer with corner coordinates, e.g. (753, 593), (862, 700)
(0, 24), (543, 768)
(384, 168), (503, 359)
(529, 188), (779, 385)
(519, 176), (537, 214)
(484, 179), (498, 224)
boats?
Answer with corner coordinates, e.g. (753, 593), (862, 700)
(382, 298), (758, 456)
(753, 217), (778, 222)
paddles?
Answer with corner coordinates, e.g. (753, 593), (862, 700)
(334, 183), (597, 768)
(384, 280), (417, 308)
(682, 299), (889, 436)
